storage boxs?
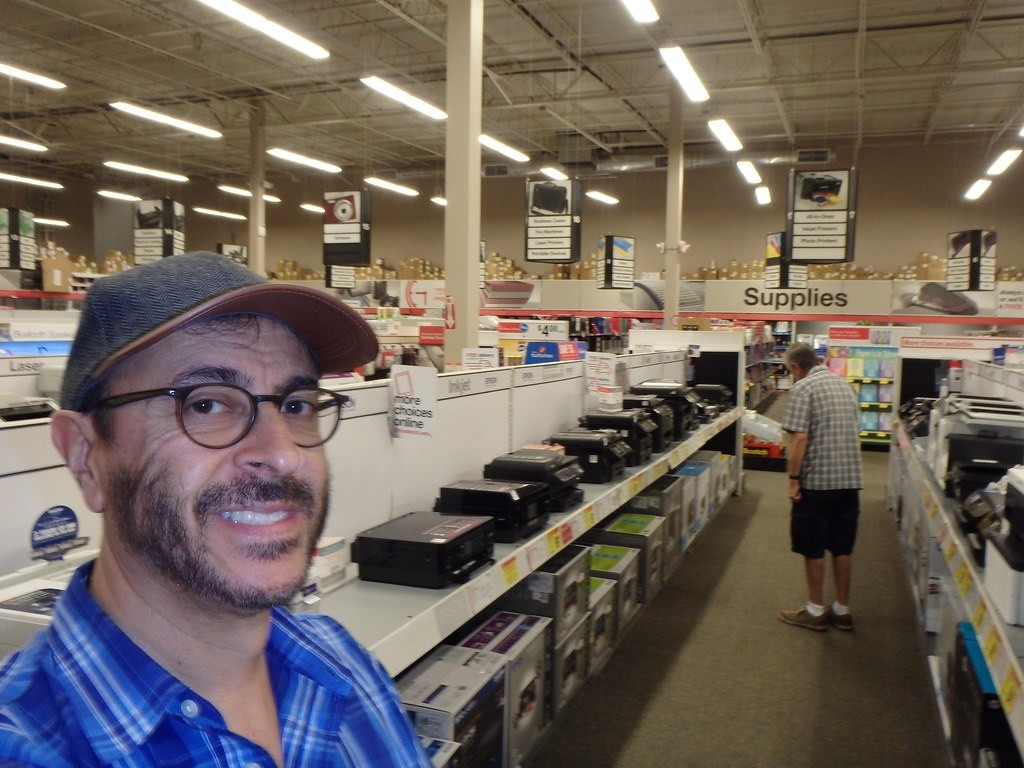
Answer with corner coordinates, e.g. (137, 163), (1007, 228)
(32, 238), (1024, 296)
(888, 443), (1024, 768)
(395, 450), (739, 768)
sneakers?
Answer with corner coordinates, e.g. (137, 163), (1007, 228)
(831, 608), (853, 630)
(778, 606), (831, 631)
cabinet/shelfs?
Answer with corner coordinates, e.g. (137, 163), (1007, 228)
(843, 377), (899, 453)
(744, 339), (775, 411)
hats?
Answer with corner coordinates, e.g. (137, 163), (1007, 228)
(60, 252), (379, 410)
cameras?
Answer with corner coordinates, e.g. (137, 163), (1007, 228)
(801, 178), (843, 200)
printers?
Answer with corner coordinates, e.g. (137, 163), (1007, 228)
(898, 395), (1024, 626)
(350, 380), (733, 590)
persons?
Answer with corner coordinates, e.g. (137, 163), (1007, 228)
(0, 250), (432, 768)
(777, 340), (862, 632)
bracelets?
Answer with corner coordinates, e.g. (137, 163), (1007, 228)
(788, 475), (801, 480)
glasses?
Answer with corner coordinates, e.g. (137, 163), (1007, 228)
(82, 383), (349, 448)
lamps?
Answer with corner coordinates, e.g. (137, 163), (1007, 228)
(0, 0), (1024, 226)
